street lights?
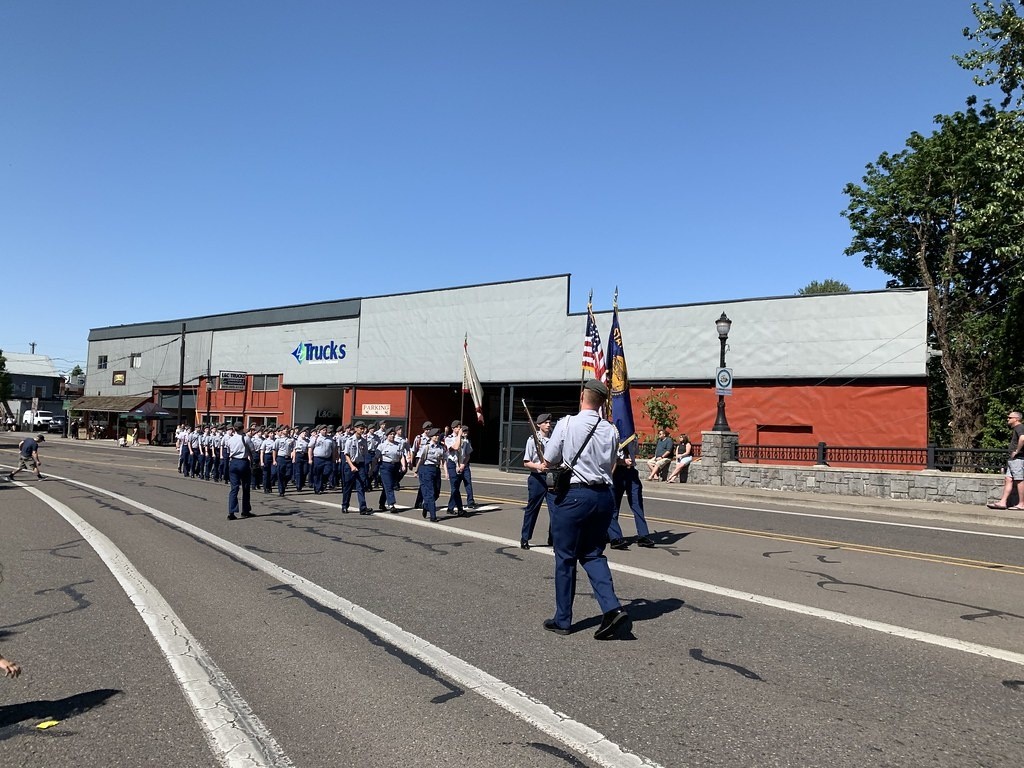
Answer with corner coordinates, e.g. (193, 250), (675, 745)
(205, 381), (213, 424)
(61, 380), (71, 438)
(712, 311), (732, 432)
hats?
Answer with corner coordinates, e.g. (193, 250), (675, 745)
(585, 379), (608, 399)
(461, 426), (468, 431)
(451, 420), (461, 428)
(423, 421), (432, 428)
(37, 435), (45, 442)
(428, 429), (440, 437)
(534, 414), (552, 424)
(184, 420), (403, 436)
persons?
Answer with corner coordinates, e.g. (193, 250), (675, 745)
(227, 421), (256, 520)
(647, 430), (674, 480)
(70, 418), (95, 440)
(1, 413), (16, 432)
(520, 413), (655, 550)
(8, 434), (49, 481)
(119, 420), (163, 448)
(665, 433), (693, 483)
(0, 654), (22, 679)
(987, 411), (1024, 510)
(174, 419), (479, 519)
(542, 379), (629, 641)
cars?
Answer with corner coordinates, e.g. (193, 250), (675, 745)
(46, 416), (69, 433)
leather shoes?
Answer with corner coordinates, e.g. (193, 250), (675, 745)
(543, 618), (571, 635)
(422, 503), (427, 519)
(342, 507), (349, 514)
(379, 505), (398, 513)
(611, 537), (633, 550)
(446, 508), (457, 515)
(548, 539), (554, 545)
(430, 517), (439, 522)
(457, 509), (468, 517)
(593, 607), (630, 639)
(468, 502), (479, 508)
(521, 538), (530, 550)
(414, 504), (422, 509)
(638, 537), (658, 547)
(360, 508), (372, 515)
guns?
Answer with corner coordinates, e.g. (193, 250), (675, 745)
(521, 398), (544, 463)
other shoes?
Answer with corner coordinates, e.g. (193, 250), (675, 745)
(644, 477), (659, 481)
(177, 469), (229, 484)
(7, 475), (16, 482)
(227, 511), (258, 520)
(37, 476), (49, 481)
(667, 479), (676, 484)
(251, 480), (339, 497)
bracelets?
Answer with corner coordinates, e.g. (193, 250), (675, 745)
(1015, 450), (1019, 453)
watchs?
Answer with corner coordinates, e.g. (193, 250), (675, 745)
(661, 456), (663, 459)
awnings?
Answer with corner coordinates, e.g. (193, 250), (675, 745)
(69, 396), (152, 413)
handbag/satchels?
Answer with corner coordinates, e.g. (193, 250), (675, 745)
(546, 465), (571, 494)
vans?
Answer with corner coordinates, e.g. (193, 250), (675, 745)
(23, 410), (53, 426)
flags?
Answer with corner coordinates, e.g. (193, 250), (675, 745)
(581, 303), (607, 419)
(462, 344), (485, 426)
(605, 304), (638, 467)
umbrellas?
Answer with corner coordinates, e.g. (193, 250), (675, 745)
(119, 402), (187, 421)
